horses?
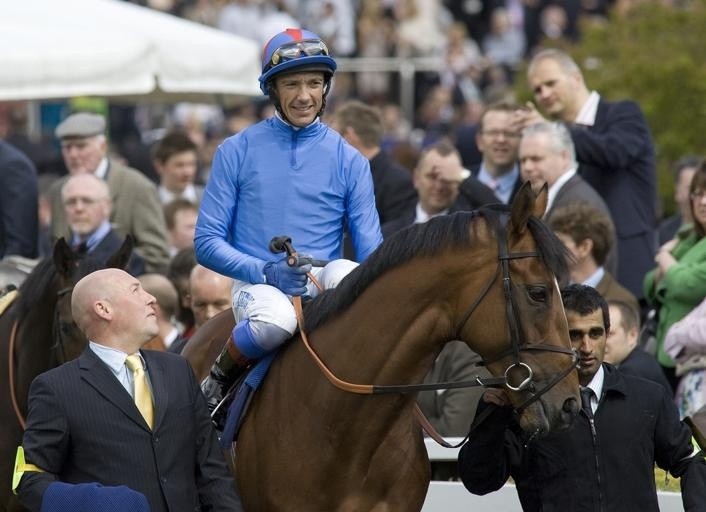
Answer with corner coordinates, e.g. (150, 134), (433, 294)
(177, 179), (582, 512)
(0, 234), (166, 511)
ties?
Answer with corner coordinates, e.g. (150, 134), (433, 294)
(125, 354), (155, 428)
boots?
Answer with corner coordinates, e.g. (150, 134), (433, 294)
(200, 333), (261, 432)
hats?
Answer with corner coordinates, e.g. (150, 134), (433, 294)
(55, 112), (107, 140)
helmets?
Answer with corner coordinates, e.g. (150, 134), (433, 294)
(258, 27), (335, 95)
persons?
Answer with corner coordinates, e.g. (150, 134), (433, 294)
(13, 268), (244, 512)
(193, 28), (385, 408)
(0, 113), (234, 354)
(218, 0), (617, 109)
(333, 49), (706, 512)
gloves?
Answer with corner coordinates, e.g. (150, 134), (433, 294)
(263, 254), (312, 296)
